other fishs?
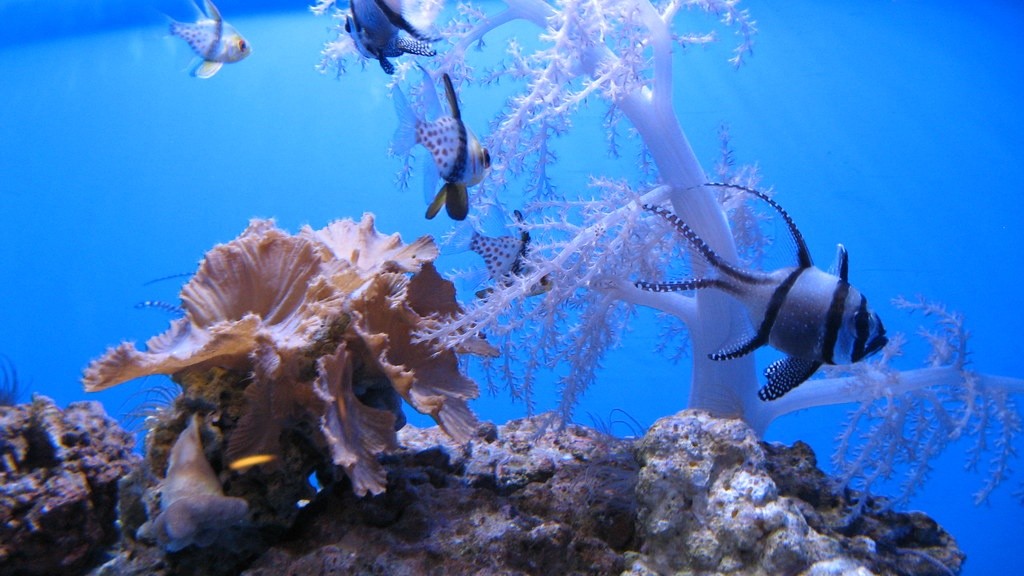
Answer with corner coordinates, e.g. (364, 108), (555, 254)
(343, 1), (556, 302)
(633, 182), (891, 402)
(161, 1), (254, 79)
